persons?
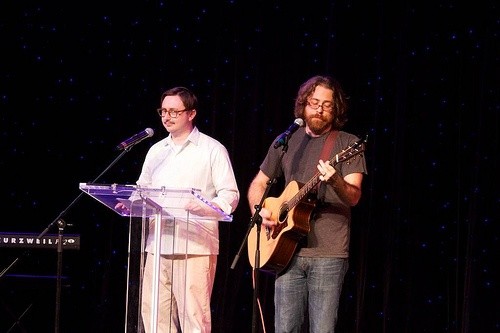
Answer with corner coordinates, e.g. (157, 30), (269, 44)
(247, 76), (368, 333)
(114, 88), (240, 332)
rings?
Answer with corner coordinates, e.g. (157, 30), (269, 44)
(323, 172), (327, 177)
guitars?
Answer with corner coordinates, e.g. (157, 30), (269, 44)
(248, 134), (369, 274)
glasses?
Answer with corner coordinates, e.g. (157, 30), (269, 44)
(157, 108), (190, 118)
(306, 98), (337, 112)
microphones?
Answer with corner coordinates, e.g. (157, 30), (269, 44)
(273, 118), (304, 149)
(116, 127), (154, 150)
